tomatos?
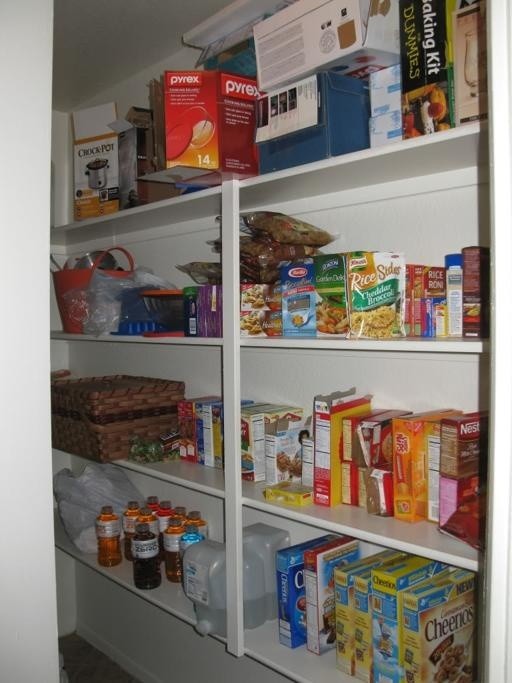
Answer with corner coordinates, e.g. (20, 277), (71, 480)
(403, 108), (415, 133)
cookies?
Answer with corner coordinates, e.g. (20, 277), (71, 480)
(434, 644), (474, 682)
(292, 458), (301, 479)
(276, 449), (292, 472)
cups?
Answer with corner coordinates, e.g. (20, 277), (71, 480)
(463, 26), (488, 96)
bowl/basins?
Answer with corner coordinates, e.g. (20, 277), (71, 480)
(142, 290), (185, 329)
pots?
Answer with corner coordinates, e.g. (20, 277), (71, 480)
(85, 159), (110, 189)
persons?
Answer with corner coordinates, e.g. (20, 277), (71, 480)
(294, 430), (310, 458)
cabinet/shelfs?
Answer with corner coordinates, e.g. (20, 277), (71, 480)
(51, 120), (489, 683)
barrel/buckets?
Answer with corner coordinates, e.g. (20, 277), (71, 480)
(183, 540), (267, 638)
(245, 520), (291, 622)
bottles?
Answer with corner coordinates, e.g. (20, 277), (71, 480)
(318, 8), (357, 54)
(98, 496), (208, 590)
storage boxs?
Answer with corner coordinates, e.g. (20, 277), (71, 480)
(255, 0), (488, 176)
(71, 68), (256, 221)
